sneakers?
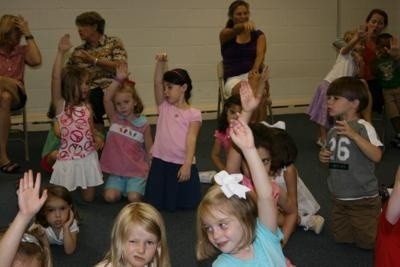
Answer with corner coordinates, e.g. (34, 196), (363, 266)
(305, 214), (324, 235)
(0, 157), (20, 174)
(315, 134), (327, 148)
(390, 132), (400, 148)
(197, 170), (217, 185)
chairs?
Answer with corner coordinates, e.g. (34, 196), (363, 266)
(216, 59), (275, 126)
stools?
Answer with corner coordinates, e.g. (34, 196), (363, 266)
(7, 106), (30, 162)
